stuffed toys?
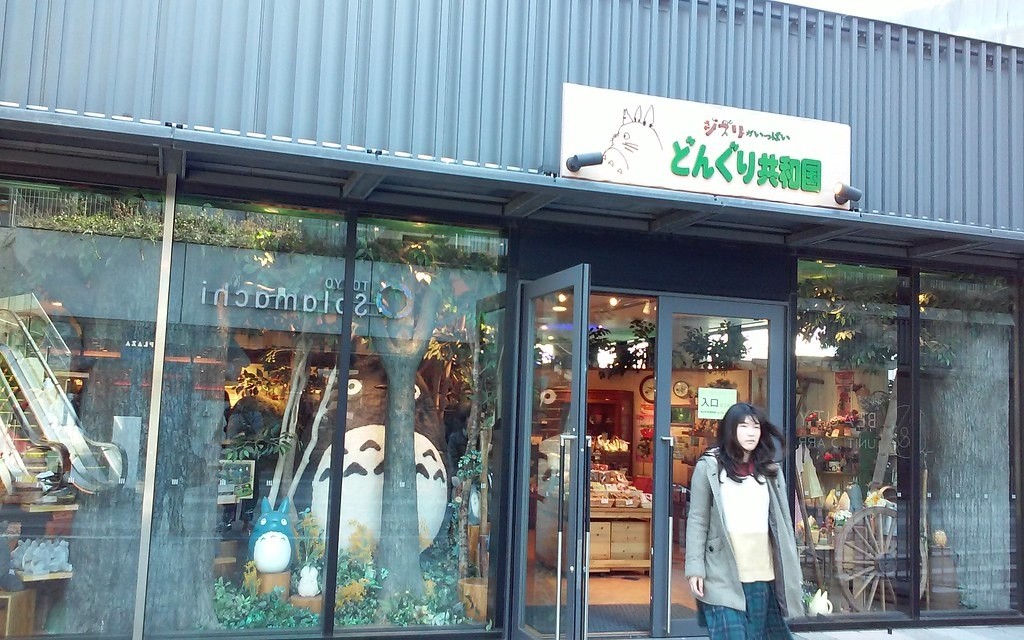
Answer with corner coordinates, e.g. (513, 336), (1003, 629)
(302, 345), (454, 574)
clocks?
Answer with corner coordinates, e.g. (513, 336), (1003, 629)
(673, 381), (689, 397)
(639, 374), (655, 403)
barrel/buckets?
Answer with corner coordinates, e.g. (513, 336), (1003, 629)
(458, 576), (488, 620)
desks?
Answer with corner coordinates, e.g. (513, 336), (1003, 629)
(796, 542), (855, 613)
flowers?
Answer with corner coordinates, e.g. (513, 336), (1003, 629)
(866, 492), (887, 508)
(828, 446), (844, 460)
(846, 408), (860, 427)
(834, 510), (852, 526)
(830, 415), (846, 424)
(805, 413), (819, 427)
(796, 515), (818, 531)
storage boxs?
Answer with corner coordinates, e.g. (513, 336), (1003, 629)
(611, 518), (650, 575)
(535, 510), (610, 575)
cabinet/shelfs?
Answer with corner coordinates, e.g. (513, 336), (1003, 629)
(797, 433), (857, 474)
(532, 390), (572, 431)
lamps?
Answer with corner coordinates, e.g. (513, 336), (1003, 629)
(834, 182), (861, 206)
(566, 152), (605, 172)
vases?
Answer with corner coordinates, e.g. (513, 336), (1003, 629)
(803, 530), (820, 545)
(831, 423), (845, 435)
(870, 514), (874, 536)
(828, 462), (841, 471)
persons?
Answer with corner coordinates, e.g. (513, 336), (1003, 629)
(147, 366), (195, 539)
(683, 402), (805, 640)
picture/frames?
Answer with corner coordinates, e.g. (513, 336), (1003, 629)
(835, 385), (852, 417)
(834, 371), (854, 386)
